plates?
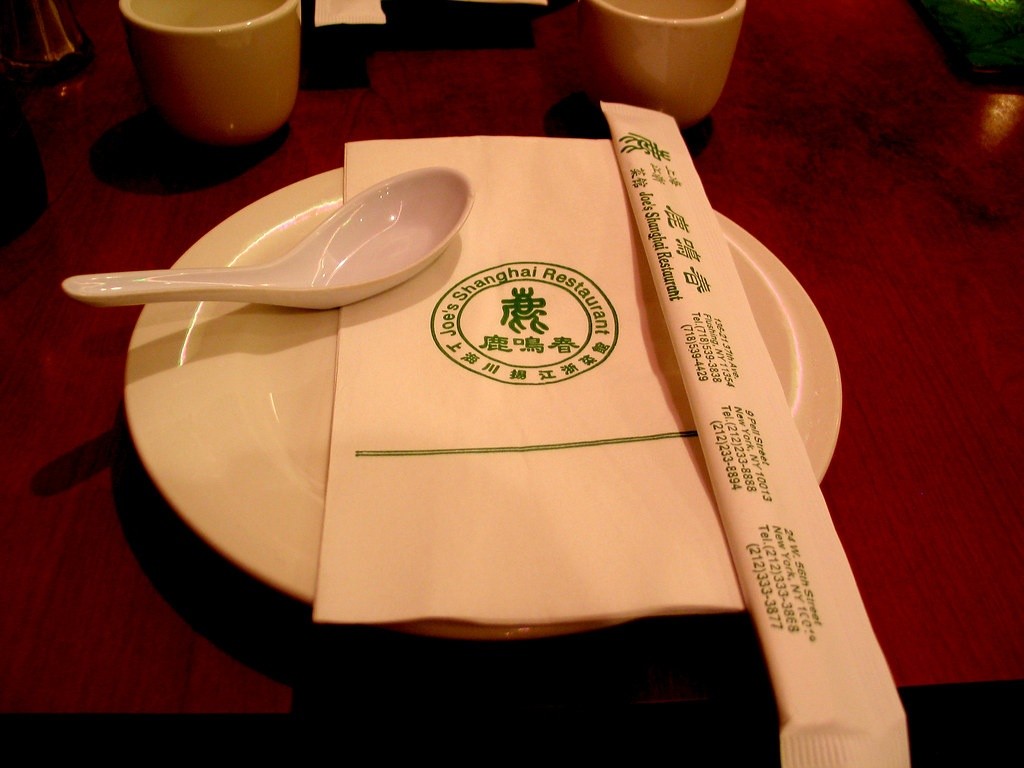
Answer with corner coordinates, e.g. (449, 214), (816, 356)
(123, 166), (842, 640)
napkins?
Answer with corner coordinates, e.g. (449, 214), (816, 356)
(312, 135), (745, 625)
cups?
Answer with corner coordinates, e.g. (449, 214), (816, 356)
(118, 0), (302, 144)
(579, 0), (747, 128)
(0, 0), (90, 87)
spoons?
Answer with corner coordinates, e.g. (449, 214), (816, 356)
(62, 166), (474, 310)
